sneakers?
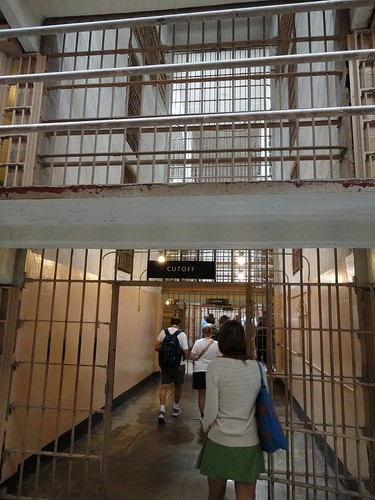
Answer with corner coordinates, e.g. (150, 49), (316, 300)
(172, 408), (182, 416)
(200, 417), (204, 423)
(158, 411), (166, 424)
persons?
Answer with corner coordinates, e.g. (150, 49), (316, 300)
(202, 313), (267, 366)
(153, 316), (183, 421)
(199, 320), (266, 500)
(191, 323), (220, 418)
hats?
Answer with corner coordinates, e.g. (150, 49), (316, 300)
(171, 317), (181, 324)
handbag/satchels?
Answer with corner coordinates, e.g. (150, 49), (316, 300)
(255, 362), (288, 453)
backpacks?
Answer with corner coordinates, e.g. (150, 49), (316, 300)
(158, 328), (184, 368)
